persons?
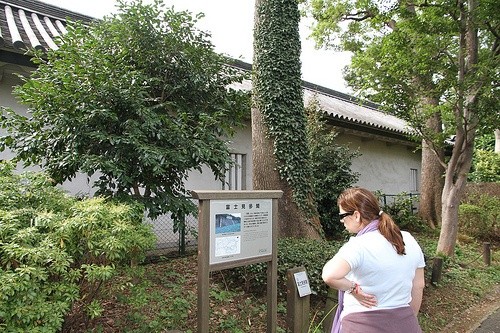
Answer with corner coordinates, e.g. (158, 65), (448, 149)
(322, 187), (426, 333)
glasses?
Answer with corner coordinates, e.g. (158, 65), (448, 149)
(338, 212), (353, 219)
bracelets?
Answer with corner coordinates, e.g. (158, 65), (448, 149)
(345, 282), (360, 295)
